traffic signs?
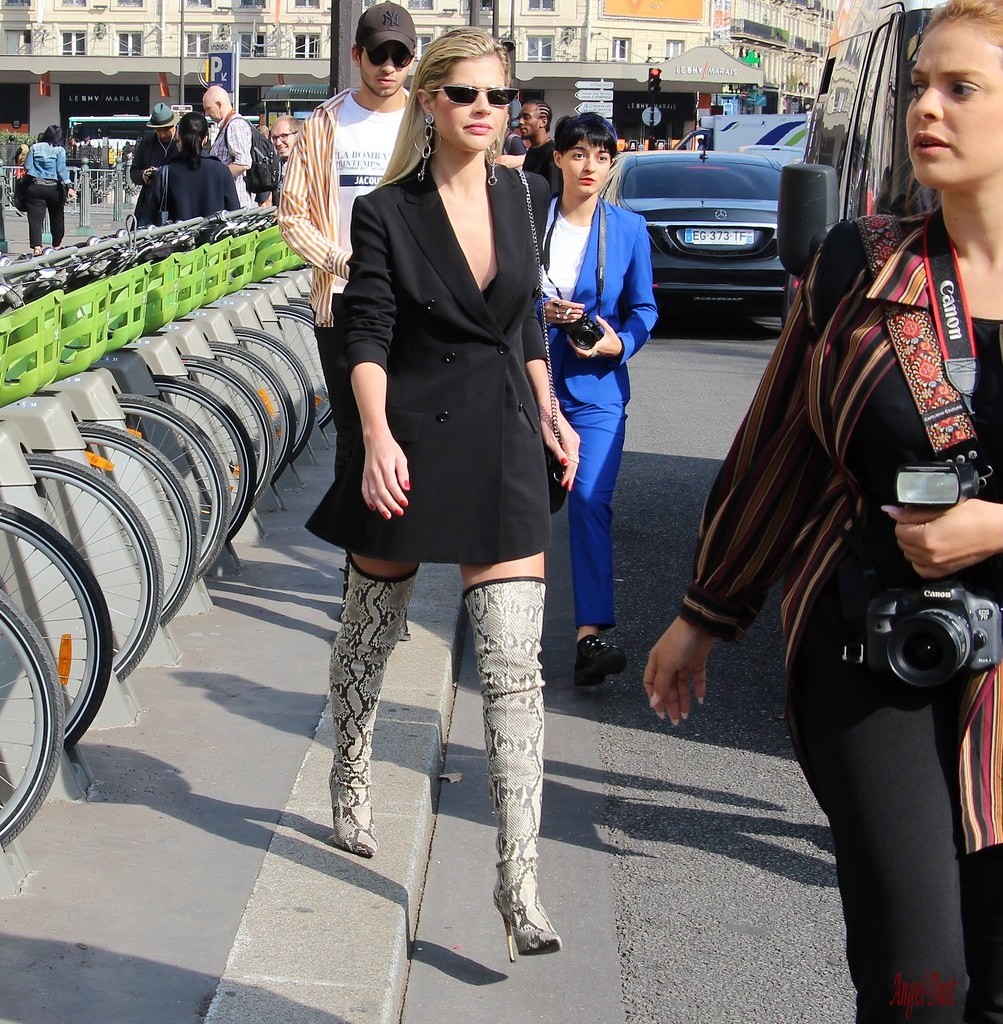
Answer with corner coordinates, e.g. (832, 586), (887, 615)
(574, 79), (613, 124)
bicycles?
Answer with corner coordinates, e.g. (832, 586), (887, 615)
(0, 202), (332, 849)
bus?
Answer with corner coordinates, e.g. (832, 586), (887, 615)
(68, 114), (259, 179)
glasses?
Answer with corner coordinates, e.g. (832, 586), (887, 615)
(270, 131), (298, 144)
(361, 45), (413, 68)
(428, 85), (519, 107)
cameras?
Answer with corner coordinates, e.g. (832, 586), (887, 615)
(558, 312), (604, 350)
(867, 462), (1002, 689)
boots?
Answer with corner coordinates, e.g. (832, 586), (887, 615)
(462, 577), (562, 963)
(328, 551), (421, 859)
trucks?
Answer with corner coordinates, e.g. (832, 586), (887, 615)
(673, 114), (807, 152)
(777, 1), (937, 279)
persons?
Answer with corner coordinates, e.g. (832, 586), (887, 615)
(15, 135), (137, 216)
(23, 124), (76, 258)
(536, 111), (659, 687)
(133, 85), (303, 207)
(697, 139), (705, 151)
(278, 1), (417, 640)
(131, 111), (241, 232)
(496, 97), (565, 201)
(304, 30), (580, 966)
(642, 0), (1002, 1024)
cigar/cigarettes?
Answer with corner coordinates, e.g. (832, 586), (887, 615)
(566, 307), (573, 317)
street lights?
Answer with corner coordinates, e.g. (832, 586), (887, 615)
(798, 82), (804, 113)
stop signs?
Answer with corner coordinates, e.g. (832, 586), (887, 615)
(741, 92), (746, 99)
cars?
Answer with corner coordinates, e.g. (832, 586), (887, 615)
(600, 145), (807, 336)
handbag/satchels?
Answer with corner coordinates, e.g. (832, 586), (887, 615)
(152, 165), (174, 258)
(13, 177), (29, 211)
(543, 448), (568, 515)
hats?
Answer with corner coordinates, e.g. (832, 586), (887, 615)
(146, 102), (180, 128)
(356, 0), (417, 56)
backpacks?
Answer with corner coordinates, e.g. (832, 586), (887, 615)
(224, 117), (282, 193)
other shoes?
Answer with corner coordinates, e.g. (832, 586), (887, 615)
(54, 247), (64, 250)
(34, 247), (43, 256)
(574, 635), (625, 686)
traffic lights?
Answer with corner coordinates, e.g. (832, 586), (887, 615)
(648, 68), (662, 95)
(650, 133), (658, 144)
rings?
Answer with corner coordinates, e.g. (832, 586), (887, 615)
(565, 449), (581, 464)
(553, 300), (562, 320)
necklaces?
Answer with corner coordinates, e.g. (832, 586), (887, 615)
(158, 136), (172, 158)
